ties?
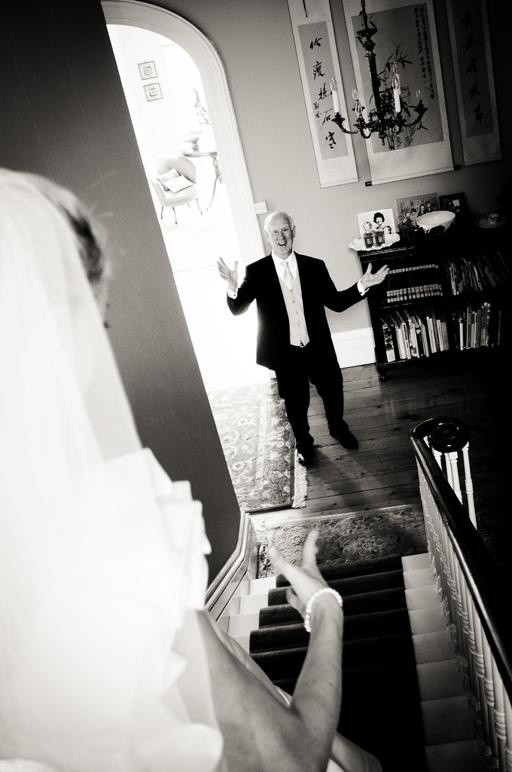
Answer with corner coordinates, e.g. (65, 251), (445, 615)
(283, 262), (295, 292)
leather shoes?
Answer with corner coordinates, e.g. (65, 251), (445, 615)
(295, 424), (360, 468)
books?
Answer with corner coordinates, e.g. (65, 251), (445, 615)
(374, 247), (511, 362)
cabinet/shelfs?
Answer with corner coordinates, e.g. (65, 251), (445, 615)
(357, 215), (506, 383)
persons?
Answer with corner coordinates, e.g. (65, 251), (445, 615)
(373, 212), (391, 234)
(0, 164), (386, 772)
(362, 221), (373, 234)
(400, 200), (433, 224)
(215, 208), (392, 469)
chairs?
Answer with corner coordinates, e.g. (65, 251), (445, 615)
(150, 157), (203, 225)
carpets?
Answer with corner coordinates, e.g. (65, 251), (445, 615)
(206, 377), (295, 513)
(256, 503), (429, 579)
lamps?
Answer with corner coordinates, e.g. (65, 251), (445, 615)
(327, 1), (426, 146)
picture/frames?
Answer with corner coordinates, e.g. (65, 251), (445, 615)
(358, 208), (396, 241)
(137, 60), (164, 103)
(396, 191), (469, 231)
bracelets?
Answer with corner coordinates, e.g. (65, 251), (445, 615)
(303, 585), (344, 633)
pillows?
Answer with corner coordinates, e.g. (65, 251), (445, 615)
(156, 168), (193, 194)
